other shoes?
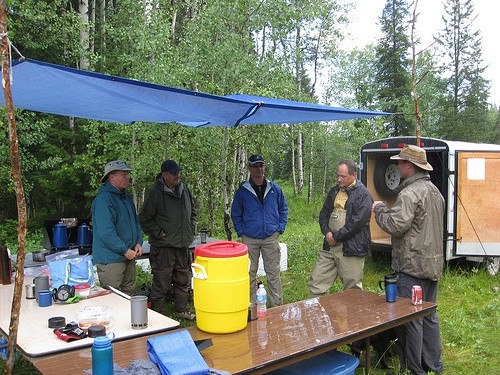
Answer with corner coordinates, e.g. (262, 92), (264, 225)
(175, 311), (196, 321)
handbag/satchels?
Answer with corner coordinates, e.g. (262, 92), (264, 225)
(48, 252), (96, 290)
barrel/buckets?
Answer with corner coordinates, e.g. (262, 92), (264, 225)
(190, 239), (252, 334)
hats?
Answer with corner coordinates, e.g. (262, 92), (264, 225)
(248, 155), (264, 164)
(100, 161), (134, 182)
(161, 160), (184, 173)
(389, 145), (434, 171)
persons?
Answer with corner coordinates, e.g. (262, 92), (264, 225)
(372, 145), (446, 375)
(231, 154), (287, 307)
(308, 159), (373, 299)
(91, 161), (144, 297)
(137, 160), (197, 321)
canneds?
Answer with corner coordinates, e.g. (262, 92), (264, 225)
(412, 285), (423, 305)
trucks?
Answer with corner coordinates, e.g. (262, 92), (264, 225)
(360, 136), (500, 276)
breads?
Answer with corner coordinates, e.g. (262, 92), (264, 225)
(79, 320), (110, 328)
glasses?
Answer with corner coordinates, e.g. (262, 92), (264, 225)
(251, 165), (264, 168)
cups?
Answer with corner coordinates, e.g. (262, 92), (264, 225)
(26, 284), (36, 299)
(201, 228), (207, 243)
(37, 290), (52, 307)
(33, 275), (49, 301)
(247, 302), (258, 320)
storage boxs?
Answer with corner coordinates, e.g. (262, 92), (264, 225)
(273, 350), (359, 375)
(257, 243), (288, 276)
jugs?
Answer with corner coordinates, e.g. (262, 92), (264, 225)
(51, 219), (72, 248)
(76, 223), (93, 248)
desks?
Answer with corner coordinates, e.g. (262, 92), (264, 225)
(10, 235), (221, 269)
(0, 272), (180, 357)
(32, 289), (437, 375)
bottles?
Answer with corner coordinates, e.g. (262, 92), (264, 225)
(379, 272), (399, 303)
(129, 296), (149, 329)
(255, 284), (268, 318)
(91, 331), (116, 375)
